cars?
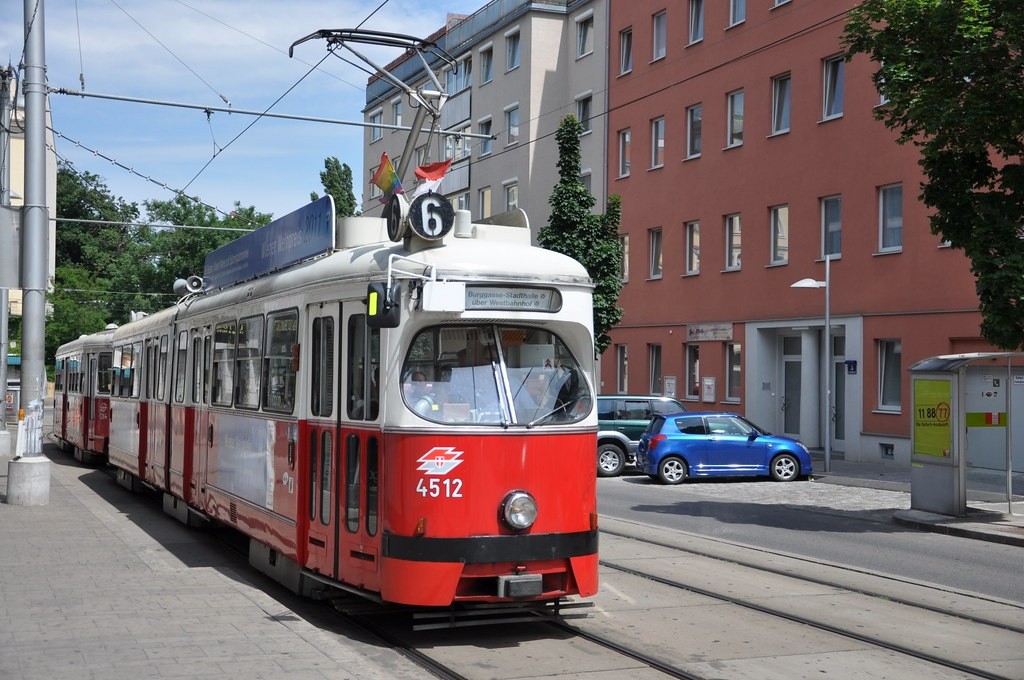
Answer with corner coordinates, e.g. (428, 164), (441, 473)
(635, 411), (813, 486)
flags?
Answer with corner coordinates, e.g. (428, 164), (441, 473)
(413, 159), (452, 197)
(368, 151), (405, 204)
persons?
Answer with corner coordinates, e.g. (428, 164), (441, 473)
(466, 349), (546, 414)
(404, 364), (467, 407)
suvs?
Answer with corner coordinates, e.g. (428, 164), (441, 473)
(568, 395), (738, 477)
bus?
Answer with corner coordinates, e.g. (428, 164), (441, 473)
(108, 195), (600, 636)
(53, 310), (150, 464)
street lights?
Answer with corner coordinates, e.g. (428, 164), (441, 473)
(790, 255), (832, 472)
